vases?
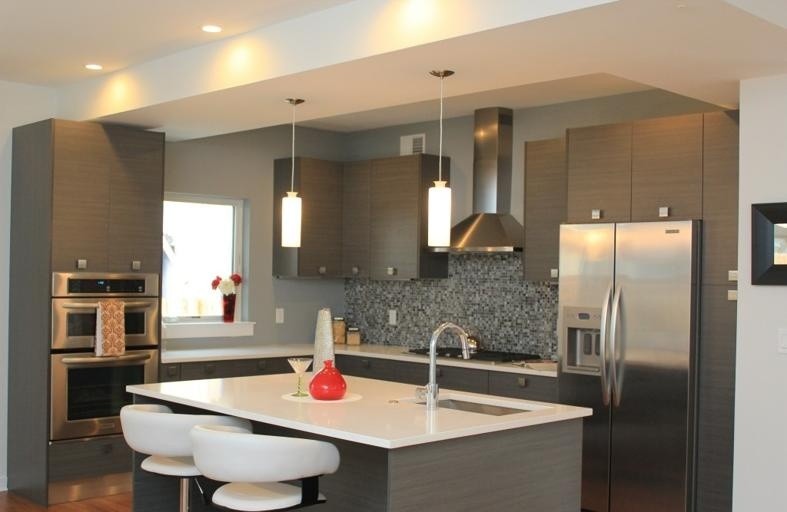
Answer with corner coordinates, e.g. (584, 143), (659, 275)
(309, 361), (347, 401)
(223, 295), (236, 321)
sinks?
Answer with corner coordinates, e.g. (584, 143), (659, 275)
(398, 391), (555, 418)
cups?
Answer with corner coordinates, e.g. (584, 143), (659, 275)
(287, 356), (314, 397)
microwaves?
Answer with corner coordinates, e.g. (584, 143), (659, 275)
(50, 270), (161, 347)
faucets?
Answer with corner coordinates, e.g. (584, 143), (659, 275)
(413, 321), (472, 409)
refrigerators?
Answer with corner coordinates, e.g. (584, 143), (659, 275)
(557, 219), (704, 511)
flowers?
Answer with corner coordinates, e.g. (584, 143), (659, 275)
(211, 274), (242, 317)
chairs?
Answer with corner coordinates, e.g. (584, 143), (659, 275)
(119, 403), (252, 512)
(191, 423), (341, 512)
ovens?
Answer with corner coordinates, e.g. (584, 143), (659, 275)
(50, 350), (160, 442)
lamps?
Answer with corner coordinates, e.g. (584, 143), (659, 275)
(279, 97), (304, 249)
(426, 71), (455, 255)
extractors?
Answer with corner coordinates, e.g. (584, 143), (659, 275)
(422, 106), (525, 256)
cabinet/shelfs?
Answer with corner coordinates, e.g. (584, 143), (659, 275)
(524, 138), (568, 281)
(693, 279), (740, 512)
(333, 354), (558, 403)
(566, 114), (702, 225)
(272, 156), (342, 280)
(701, 107), (741, 283)
(337, 154), (450, 282)
(159, 355), (314, 382)
(6, 119), (167, 508)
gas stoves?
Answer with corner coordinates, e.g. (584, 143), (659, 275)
(407, 346), (542, 364)
